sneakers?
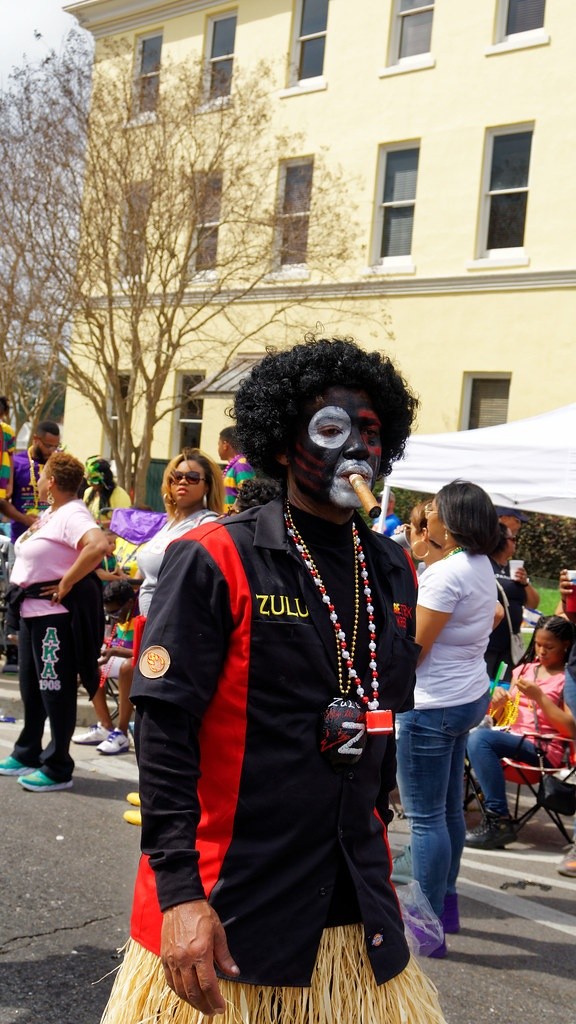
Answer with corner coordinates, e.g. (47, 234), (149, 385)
(0, 757), (38, 776)
(16, 767), (74, 792)
(70, 721), (114, 744)
(558, 840), (576, 877)
(96, 729), (130, 754)
(463, 816), (517, 848)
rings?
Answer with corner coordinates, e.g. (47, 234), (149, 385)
(53, 593), (58, 598)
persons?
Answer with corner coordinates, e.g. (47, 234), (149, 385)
(464, 612), (576, 848)
(485, 509), (540, 681)
(218, 427), (255, 517)
(370, 478), (506, 955)
(95, 338), (451, 1023)
(0, 397), (147, 793)
(227, 478), (276, 516)
(557, 569), (576, 875)
(124, 446), (224, 826)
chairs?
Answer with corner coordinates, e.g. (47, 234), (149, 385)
(461, 730), (576, 846)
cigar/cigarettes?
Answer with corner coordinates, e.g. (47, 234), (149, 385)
(348, 474), (381, 518)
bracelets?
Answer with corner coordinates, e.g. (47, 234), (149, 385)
(524, 583), (528, 587)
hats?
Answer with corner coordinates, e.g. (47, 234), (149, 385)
(495, 506), (532, 523)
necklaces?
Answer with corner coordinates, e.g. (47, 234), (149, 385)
(28, 446), (42, 508)
(284, 513), (393, 735)
(100, 614), (133, 688)
(20, 517), (53, 542)
(286, 499), (359, 693)
(446, 546), (465, 558)
(223, 454), (242, 479)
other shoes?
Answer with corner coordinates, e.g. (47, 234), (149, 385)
(439, 889), (463, 934)
(402, 909), (449, 959)
(124, 810), (142, 825)
(128, 791), (141, 806)
(3, 654), (19, 672)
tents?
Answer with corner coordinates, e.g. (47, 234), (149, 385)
(379, 402), (576, 535)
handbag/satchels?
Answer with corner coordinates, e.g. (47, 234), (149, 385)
(537, 772), (576, 817)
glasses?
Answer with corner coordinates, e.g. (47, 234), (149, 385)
(425, 502), (439, 518)
(170, 469), (206, 485)
(108, 602), (129, 618)
(227, 505), (243, 516)
(505, 535), (517, 542)
(37, 438), (60, 452)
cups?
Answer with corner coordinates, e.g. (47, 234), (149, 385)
(389, 526), (410, 549)
(509, 560), (524, 581)
(565, 570), (576, 612)
(416, 561), (426, 580)
